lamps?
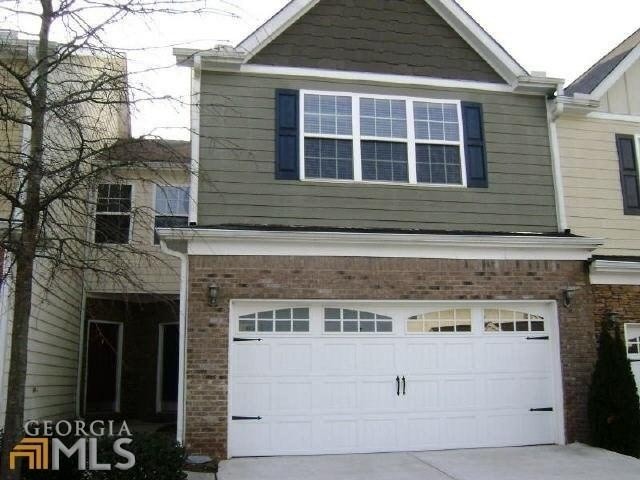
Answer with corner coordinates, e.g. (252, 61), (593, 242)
(562, 282), (577, 313)
(209, 279), (220, 308)
